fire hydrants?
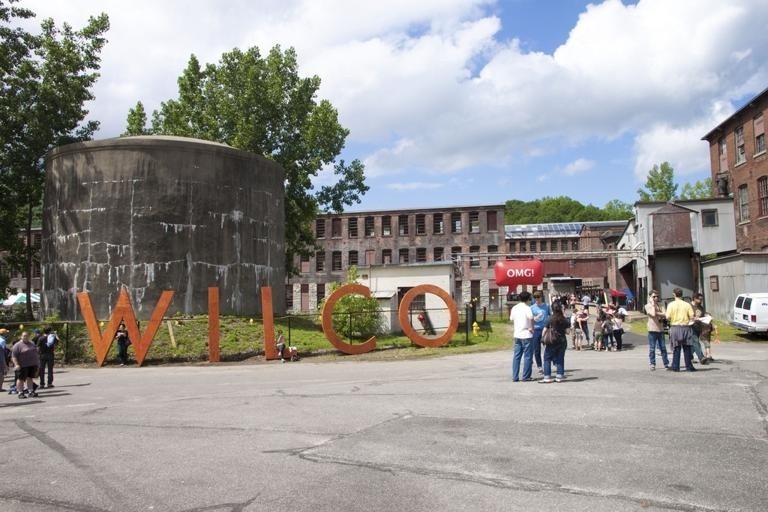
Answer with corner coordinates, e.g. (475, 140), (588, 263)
(471, 320), (481, 336)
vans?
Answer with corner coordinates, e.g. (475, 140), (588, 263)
(733, 292), (767, 340)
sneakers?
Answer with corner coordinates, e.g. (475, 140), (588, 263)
(29, 392), (38, 396)
(538, 377), (561, 382)
(18, 393), (27, 398)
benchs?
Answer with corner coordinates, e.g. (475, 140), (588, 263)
(273, 348), (297, 360)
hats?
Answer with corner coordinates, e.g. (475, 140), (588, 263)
(0, 328), (9, 334)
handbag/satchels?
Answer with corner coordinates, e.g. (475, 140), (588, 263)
(541, 327), (561, 345)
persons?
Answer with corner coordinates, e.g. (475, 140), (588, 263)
(665, 287), (698, 373)
(698, 312), (721, 362)
(551, 291), (629, 352)
(510, 291), (538, 383)
(643, 289), (670, 372)
(116, 323), (131, 367)
(0, 325), (61, 400)
(689, 294), (706, 363)
(530, 290), (551, 373)
(274, 329), (287, 363)
(537, 301), (572, 384)
